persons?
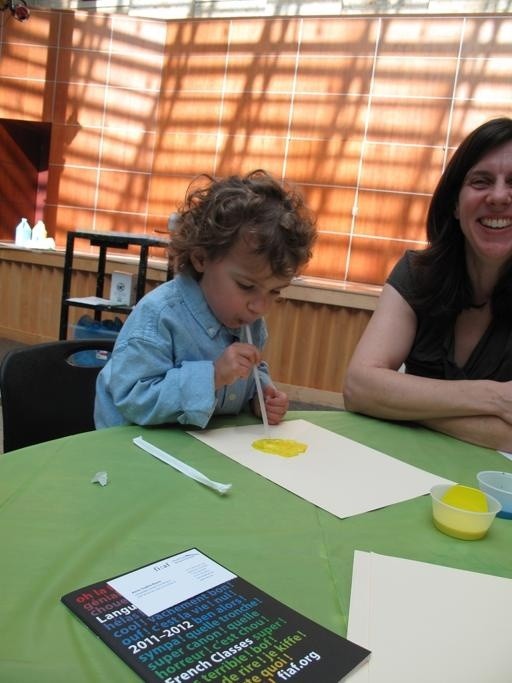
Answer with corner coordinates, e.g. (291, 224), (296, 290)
(94, 170), (319, 429)
(343, 118), (512, 453)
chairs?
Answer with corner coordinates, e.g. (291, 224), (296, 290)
(0, 337), (118, 452)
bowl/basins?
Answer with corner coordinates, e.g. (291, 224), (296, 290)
(429, 469), (512, 539)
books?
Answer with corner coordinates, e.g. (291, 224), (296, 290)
(61, 547), (372, 683)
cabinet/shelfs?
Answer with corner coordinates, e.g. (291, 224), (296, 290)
(59, 230), (174, 340)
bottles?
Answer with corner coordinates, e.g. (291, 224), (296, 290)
(15, 217), (47, 249)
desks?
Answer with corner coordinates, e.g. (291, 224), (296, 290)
(0, 411), (512, 682)
